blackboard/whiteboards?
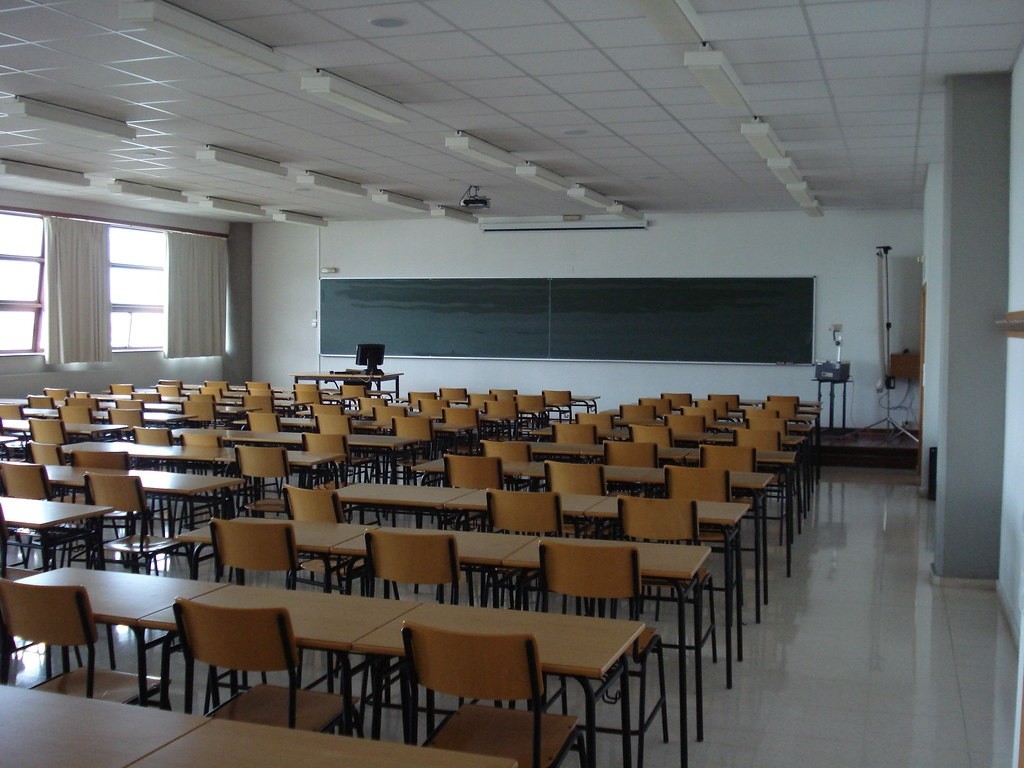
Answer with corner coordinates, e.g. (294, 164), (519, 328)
(317, 276), (816, 366)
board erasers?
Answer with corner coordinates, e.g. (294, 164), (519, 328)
(775, 361), (795, 365)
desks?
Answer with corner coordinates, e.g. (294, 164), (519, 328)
(0, 375), (854, 768)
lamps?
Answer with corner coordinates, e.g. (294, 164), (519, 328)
(0, 0), (824, 229)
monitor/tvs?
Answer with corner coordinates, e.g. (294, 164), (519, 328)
(355, 344), (385, 375)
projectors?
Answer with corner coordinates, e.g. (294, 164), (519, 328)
(463, 198), (490, 210)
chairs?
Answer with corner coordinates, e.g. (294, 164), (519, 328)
(0, 369), (800, 768)
